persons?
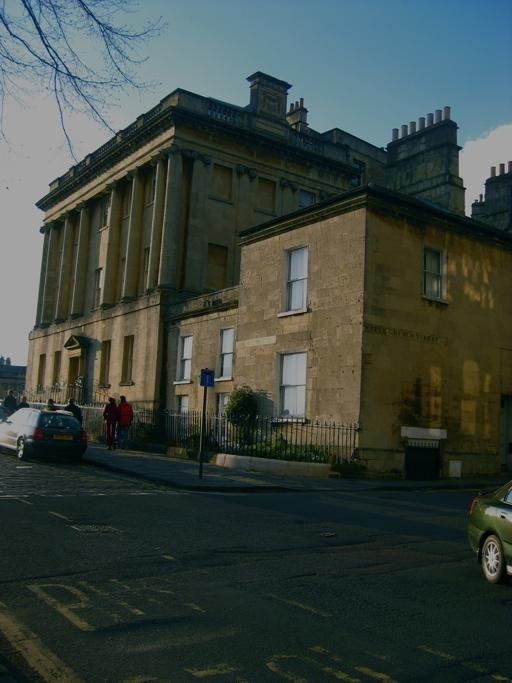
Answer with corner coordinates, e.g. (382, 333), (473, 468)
(102, 397), (118, 450)
(63, 398), (82, 424)
(46, 398), (56, 408)
(117, 394), (134, 448)
(3, 388), (18, 411)
(18, 395), (30, 408)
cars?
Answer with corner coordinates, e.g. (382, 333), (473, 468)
(0, 406), (89, 460)
(467, 479), (512, 582)
